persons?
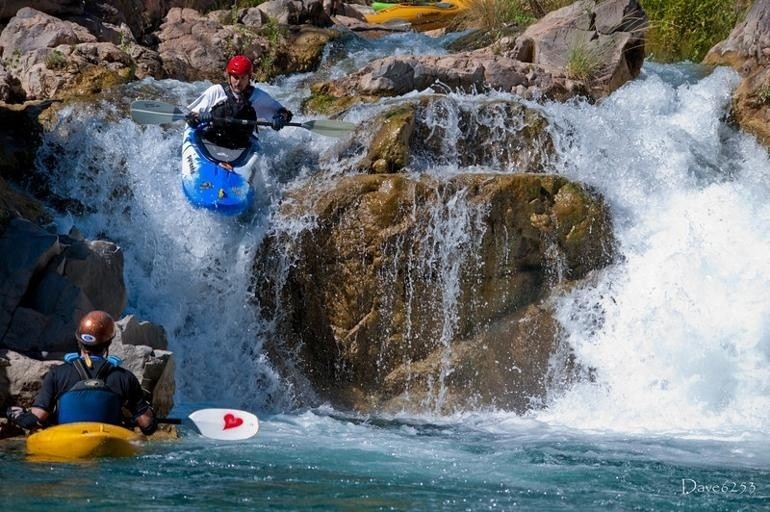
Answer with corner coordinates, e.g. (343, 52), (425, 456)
(184, 55), (294, 167)
(6, 310), (158, 435)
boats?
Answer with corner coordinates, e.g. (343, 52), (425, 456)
(26, 422), (145, 459)
(182, 122), (260, 216)
(367, 0), (473, 32)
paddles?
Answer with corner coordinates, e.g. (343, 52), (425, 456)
(157, 408), (259, 441)
(350, 28), (408, 32)
(384, 20), (438, 29)
(130, 99), (355, 137)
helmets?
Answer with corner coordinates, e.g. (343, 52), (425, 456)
(75, 310), (116, 346)
(226, 54), (254, 78)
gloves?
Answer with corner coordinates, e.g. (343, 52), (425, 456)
(184, 111), (202, 128)
(271, 108), (292, 131)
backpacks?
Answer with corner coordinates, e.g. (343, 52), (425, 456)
(57, 358), (122, 427)
(207, 82), (257, 150)
